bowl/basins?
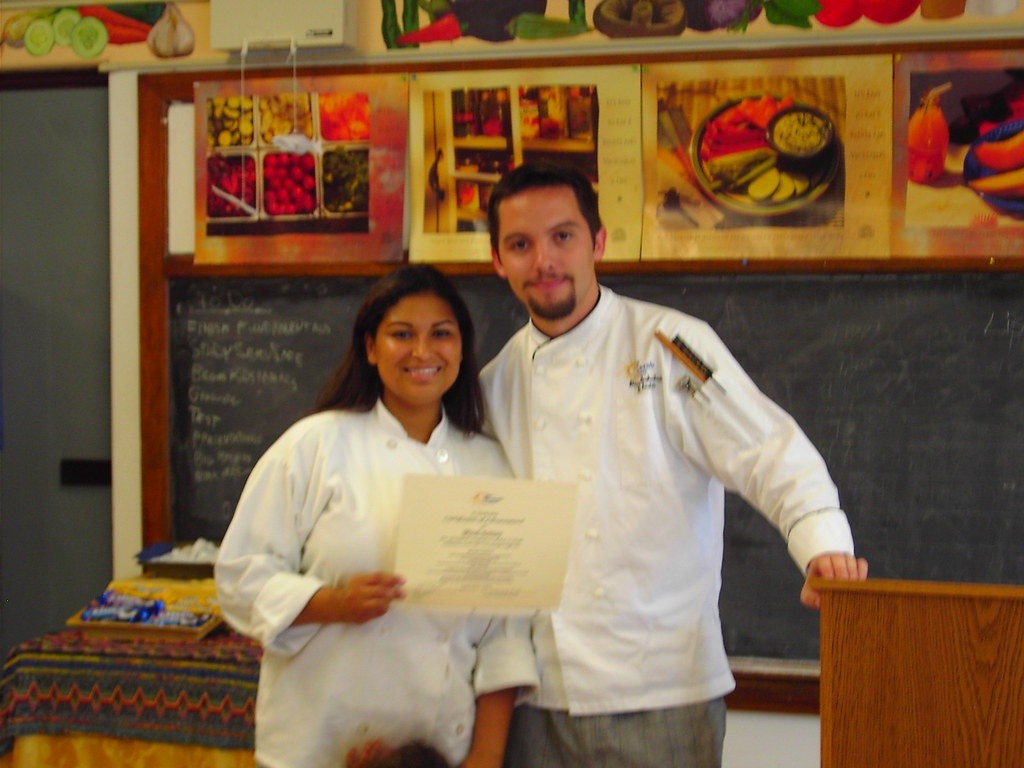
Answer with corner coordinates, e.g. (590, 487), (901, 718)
(768, 106), (834, 159)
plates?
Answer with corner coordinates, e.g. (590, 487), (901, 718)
(143, 541), (226, 579)
(963, 118), (1024, 214)
(688, 94), (840, 215)
(66, 578), (224, 641)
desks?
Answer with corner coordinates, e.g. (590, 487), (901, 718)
(0, 570), (263, 768)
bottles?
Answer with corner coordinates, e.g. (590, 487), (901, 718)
(455, 86), (596, 234)
(656, 188), (700, 231)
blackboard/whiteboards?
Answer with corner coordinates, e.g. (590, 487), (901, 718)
(158, 258), (1023, 674)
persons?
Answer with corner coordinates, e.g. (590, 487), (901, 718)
(476, 163), (867, 768)
(214, 265), (540, 768)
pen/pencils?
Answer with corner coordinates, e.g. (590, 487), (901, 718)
(655, 330), (708, 396)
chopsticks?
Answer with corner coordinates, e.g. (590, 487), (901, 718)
(656, 333), (713, 382)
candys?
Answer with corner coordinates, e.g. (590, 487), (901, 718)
(80, 590), (211, 628)
